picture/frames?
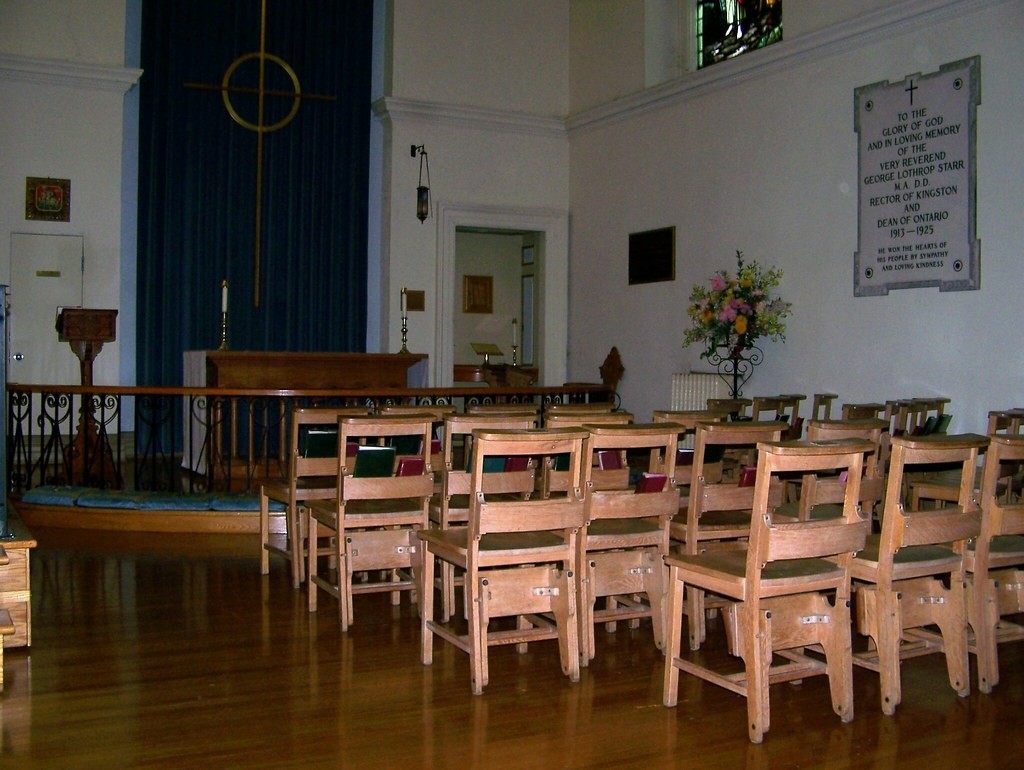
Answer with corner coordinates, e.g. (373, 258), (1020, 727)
(463, 274), (494, 314)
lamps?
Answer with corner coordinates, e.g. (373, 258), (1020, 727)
(411, 144), (434, 225)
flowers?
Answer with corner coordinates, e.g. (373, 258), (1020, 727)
(681, 248), (793, 360)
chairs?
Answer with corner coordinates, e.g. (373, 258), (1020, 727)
(259, 391), (1024, 743)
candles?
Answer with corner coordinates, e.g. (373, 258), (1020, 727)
(512, 316), (518, 346)
(222, 286), (228, 313)
(402, 293), (407, 319)
